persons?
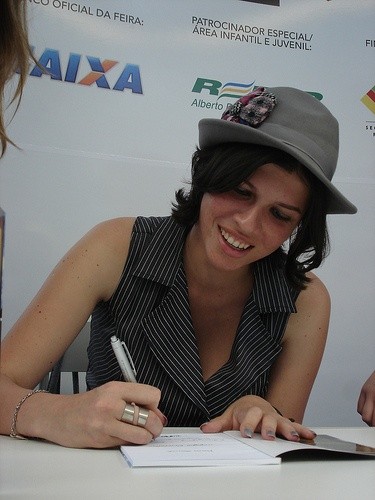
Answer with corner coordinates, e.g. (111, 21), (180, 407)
(357, 371), (375, 427)
(0, 85), (357, 450)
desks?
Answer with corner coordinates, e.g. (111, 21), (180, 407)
(0, 427), (375, 500)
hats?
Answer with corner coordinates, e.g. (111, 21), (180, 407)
(198, 86), (357, 215)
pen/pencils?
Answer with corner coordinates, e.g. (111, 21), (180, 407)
(110, 336), (155, 440)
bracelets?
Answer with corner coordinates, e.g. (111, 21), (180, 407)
(10, 389), (50, 439)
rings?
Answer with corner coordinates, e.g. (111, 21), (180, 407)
(137, 407), (149, 428)
(121, 403), (134, 425)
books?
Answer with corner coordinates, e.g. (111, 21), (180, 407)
(119, 429), (375, 468)
